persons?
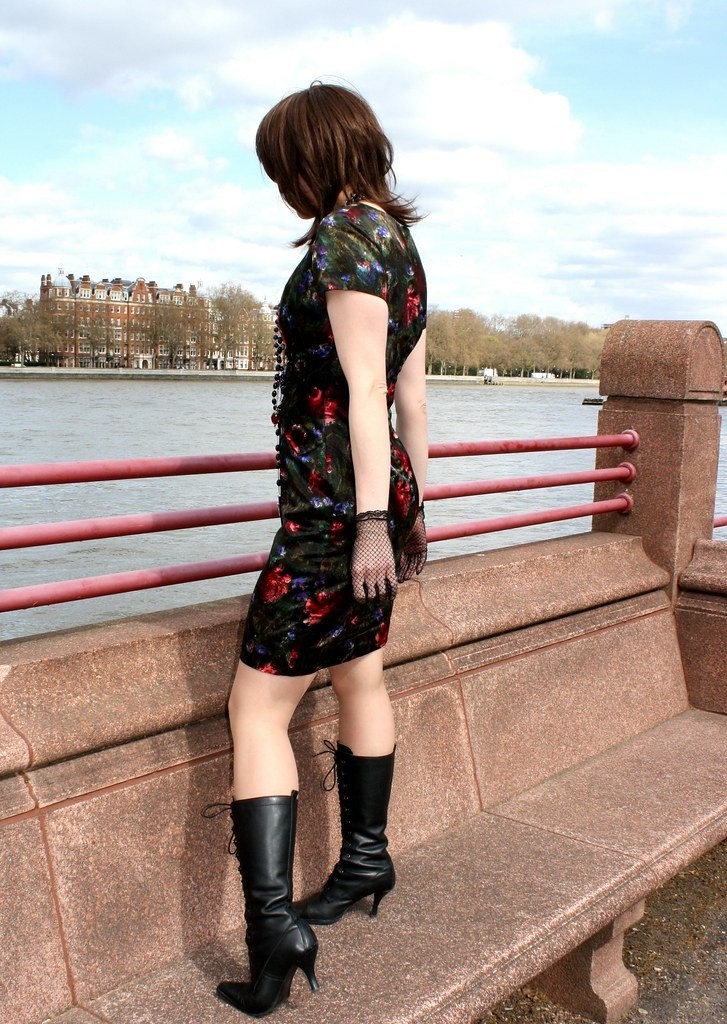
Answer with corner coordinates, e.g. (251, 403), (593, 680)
(217, 85), (427, 1017)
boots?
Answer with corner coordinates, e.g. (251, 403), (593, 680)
(292, 740), (396, 925)
(203, 790), (320, 1018)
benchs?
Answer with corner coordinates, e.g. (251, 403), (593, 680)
(1, 588), (726, 1023)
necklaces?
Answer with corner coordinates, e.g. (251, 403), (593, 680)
(272, 307), (285, 486)
